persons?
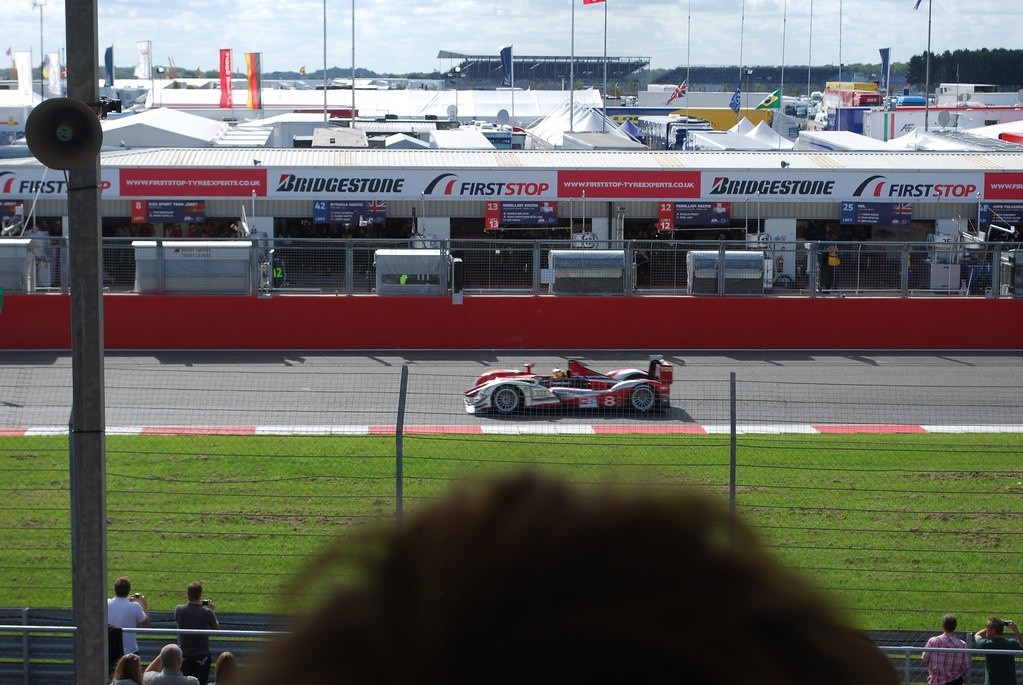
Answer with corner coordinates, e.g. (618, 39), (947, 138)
(897, 245), (914, 293)
(549, 369), (563, 387)
(273, 250), (286, 288)
(107, 451), (1023, 685)
(1, 220), (62, 235)
(170, 222), (218, 238)
(115, 220), (155, 237)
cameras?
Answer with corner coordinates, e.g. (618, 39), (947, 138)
(1003, 621), (1009, 626)
(202, 600), (208, 605)
(135, 593), (140, 599)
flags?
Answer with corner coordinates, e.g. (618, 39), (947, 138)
(104, 46), (113, 87)
(16, 52), (33, 106)
(134, 42), (153, 78)
(245, 53), (262, 109)
(583, 0), (607, 4)
(219, 49), (232, 108)
(729, 78), (741, 113)
(913, 0), (931, 10)
(47, 52), (61, 95)
(755, 88), (782, 110)
(879, 48), (889, 88)
(666, 78), (688, 105)
(500, 47), (511, 86)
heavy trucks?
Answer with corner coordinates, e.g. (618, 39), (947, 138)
(824, 89), (884, 107)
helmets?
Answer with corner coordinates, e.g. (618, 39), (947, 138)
(552, 369), (565, 379)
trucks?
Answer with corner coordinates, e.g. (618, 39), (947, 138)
(783, 91), (829, 129)
(931, 83), (997, 106)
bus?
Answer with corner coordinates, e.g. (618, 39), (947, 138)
(457, 120), (512, 151)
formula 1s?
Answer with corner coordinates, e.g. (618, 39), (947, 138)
(462, 353), (673, 414)
